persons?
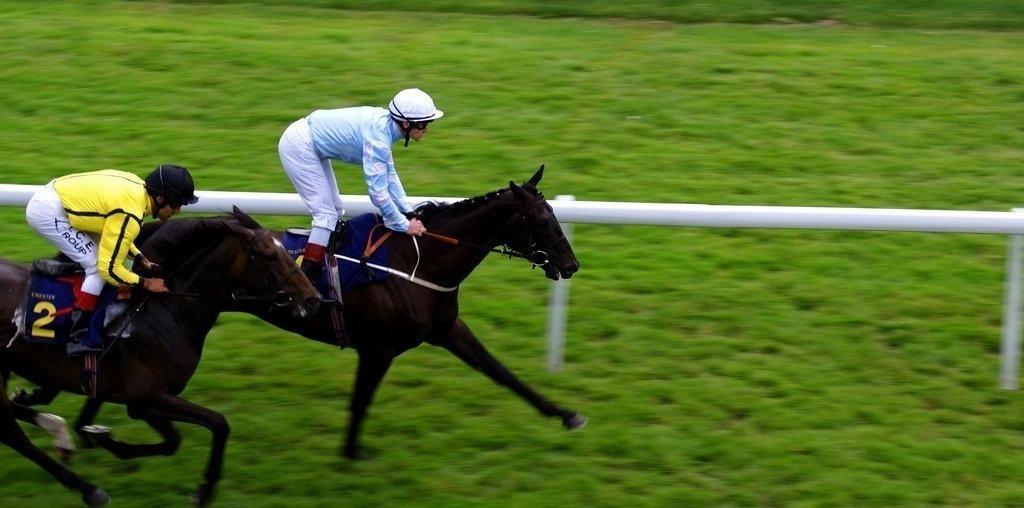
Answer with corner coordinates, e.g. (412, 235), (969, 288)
(277, 88), (444, 304)
(25, 164), (199, 352)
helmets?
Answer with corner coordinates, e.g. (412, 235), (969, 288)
(389, 88), (444, 122)
(145, 164), (198, 204)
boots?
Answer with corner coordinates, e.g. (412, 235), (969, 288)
(299, 258), (342, 306)
(64, 307), (104, 356)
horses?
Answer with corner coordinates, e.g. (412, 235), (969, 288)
(8, 164), (588, 465)
(0, 204), (324, 508)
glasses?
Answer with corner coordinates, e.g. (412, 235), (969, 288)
(414, 122), (427, 130)
(168, 200), (178, 209)
